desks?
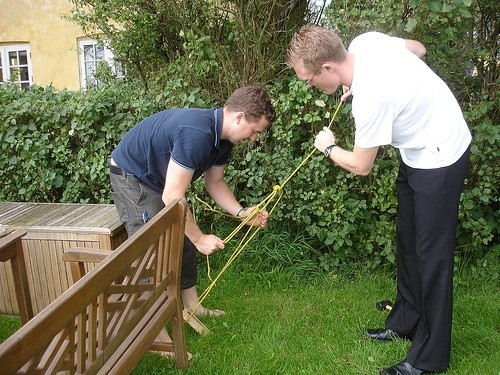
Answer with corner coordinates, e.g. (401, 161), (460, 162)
(0, 227), (34, 326)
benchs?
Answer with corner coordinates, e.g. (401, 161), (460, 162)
(0, 200), (188, 375)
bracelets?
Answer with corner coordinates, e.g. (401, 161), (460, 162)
(237, 208), (247, 222)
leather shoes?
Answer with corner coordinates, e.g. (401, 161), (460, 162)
(364, 328), (402, 342)
(380, 360), (438, 375)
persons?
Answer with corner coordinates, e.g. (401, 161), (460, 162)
(286, 24), (472, 375)
(110, 86), (276, 360)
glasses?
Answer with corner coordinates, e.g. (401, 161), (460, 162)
(304, 68), (324, 84)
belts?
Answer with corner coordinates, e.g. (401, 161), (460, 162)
(110, 166), (126, 174)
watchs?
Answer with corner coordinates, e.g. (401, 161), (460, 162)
(324, 144), (337, 159)
